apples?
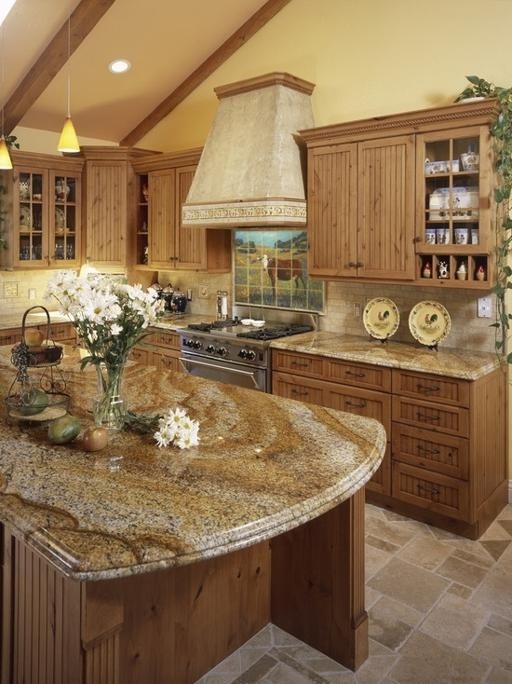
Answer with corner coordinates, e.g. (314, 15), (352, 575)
(24, 328), (44, 346)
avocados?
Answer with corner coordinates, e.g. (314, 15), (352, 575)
(20, 392), (48, 415)
(47, 416), (81, 444)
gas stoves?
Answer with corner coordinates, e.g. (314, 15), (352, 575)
(177, 318), (314, 368)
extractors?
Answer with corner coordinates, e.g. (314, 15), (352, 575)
(181, 85), (317, 229)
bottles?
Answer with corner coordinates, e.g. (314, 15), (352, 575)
(216, 291), (228, 321)
(147, 282), (187, 314)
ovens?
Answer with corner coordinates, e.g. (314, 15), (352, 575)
(177, 351), (268, 392)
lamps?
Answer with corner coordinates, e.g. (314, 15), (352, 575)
(55, 0), (81, 154)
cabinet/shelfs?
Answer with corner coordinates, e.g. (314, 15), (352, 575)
(270, 349), (390, 498)
(136, 164), (206, 270)
(306, 133), (415, 282)
(391, 368), (470, 522)
(0, 323), (75, 346)
(12, 165), (80, 267)
(85, 159), (126, 270)
(415, 124), (490, 289)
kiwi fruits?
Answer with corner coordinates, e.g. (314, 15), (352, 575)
(82, 427), (108, 453)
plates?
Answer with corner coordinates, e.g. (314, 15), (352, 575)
(20, 204), (33, 227)
(55, 206), (64, 234)
(363, 298), (400, 341)
(408, 301), (452, 346)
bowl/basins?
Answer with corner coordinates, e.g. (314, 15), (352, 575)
(240, 319), (265, 327)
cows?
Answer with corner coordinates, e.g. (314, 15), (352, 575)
(251, 253), (307, 299)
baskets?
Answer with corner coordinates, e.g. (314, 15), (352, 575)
(11, 307), (62, 365)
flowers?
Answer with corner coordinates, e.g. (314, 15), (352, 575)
(48, 264), (201, 452)
